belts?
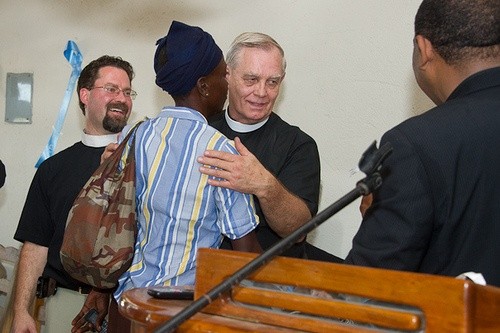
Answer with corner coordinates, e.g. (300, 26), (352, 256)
(58, 283), (91, 294)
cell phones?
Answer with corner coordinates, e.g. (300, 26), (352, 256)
(147, 288), (193, 300)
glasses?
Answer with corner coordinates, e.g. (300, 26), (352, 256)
(87, 84), (138, 101)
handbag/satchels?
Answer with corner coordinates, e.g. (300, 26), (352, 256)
(59, 119), (143, 288)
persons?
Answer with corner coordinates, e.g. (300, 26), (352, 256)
(100, 32), (320, 258)
(68, 20), (305, 333)
(8, 55), (137, 333)
(341, 1), (500, 287)
